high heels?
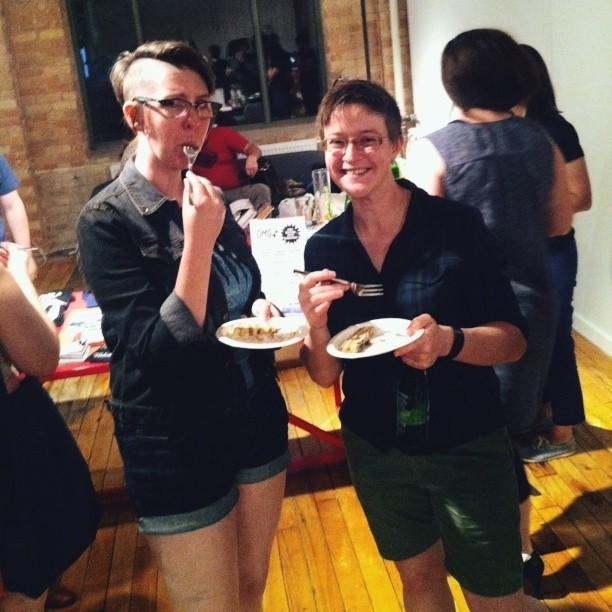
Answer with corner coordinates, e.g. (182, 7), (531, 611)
(523, 549), (546, 598)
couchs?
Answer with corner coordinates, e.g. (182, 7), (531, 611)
(88, 150), (341, 225)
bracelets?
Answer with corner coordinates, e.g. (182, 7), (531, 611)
(447, 325), (464, 360)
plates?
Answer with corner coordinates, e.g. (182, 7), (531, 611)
(326, 316), (424, 360)
(215, 316), (308, 350)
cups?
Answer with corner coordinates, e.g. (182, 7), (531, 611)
(312, 168), (331, 218)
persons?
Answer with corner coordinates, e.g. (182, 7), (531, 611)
(0, 234), (101, 612)
(297, 77), (531, 612)
(0, 152), (38, 287)
(72, 37), (293, 610)
(417, 27), (570, 459)
(510, 44), (591, 463)
(191, 124), (271, 213)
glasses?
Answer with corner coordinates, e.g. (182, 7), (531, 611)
(317, 135), (388, 151)
(132, 97), (222, 118)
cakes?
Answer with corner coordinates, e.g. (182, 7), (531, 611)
(234, 325), (297, 341)
(338, 326), (375, 352)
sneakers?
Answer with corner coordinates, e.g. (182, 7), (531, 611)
(521, 436), (576, 463)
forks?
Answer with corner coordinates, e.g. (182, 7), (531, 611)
(182, 145), (199, 207)
(294, 268), (383, 300)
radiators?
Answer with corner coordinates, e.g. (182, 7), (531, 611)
(108, 138), (319, 181)
